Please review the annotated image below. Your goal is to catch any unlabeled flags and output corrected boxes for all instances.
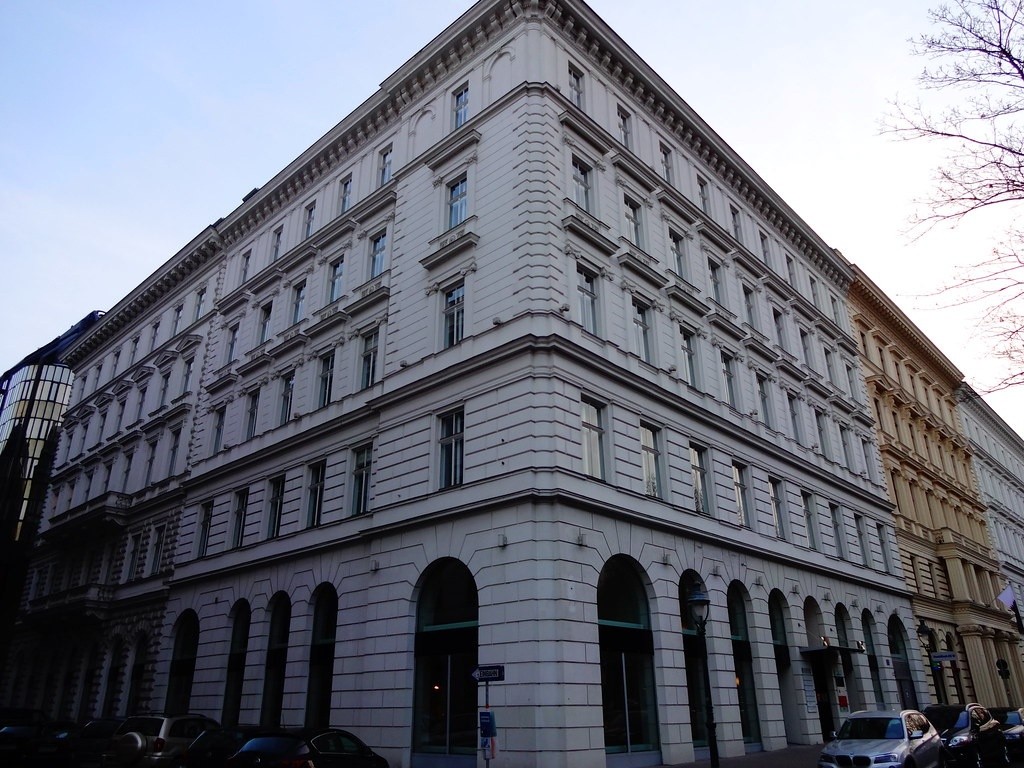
[997,586,1024,638]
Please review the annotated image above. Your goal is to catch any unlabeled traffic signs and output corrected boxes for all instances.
[470,665,506,683]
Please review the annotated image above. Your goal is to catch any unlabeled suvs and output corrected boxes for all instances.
[923,701,1009,768]
[817,708,948,768]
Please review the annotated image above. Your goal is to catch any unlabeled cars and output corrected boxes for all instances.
[988,706,1024,753]
[0,706,391,768]
